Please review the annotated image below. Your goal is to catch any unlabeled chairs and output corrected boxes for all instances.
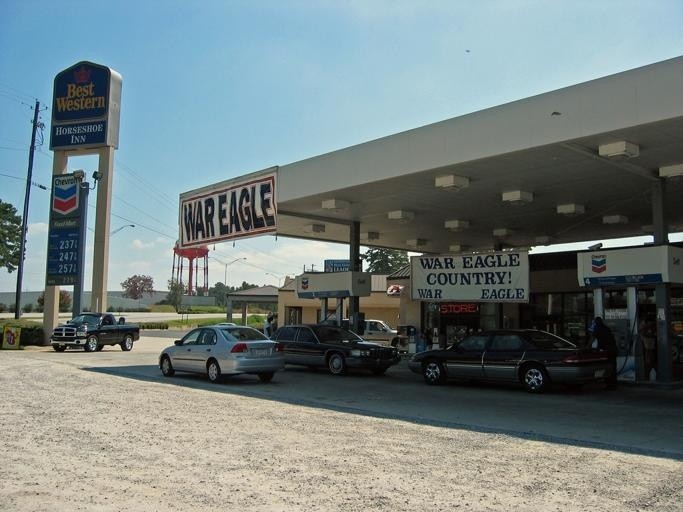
[204,335,214,344]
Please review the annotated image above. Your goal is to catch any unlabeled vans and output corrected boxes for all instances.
[334,319,401,346]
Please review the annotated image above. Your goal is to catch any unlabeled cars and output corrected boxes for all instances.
[270,324,400,375]
[409,328,606,393]
[159,323,284,384]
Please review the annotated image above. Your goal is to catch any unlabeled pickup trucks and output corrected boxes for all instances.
[49,312,139,352]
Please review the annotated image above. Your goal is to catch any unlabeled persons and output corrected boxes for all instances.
[585,317,617,391]
[263,314,273,338]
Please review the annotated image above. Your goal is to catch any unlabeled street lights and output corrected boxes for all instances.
[209,256,247,288]
[86,222,136,239]
[267,271,295,289]
[73,171,103,318]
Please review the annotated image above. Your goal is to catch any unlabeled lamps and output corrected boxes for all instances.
[660,164,682,182]
[491,191,676,246]
[435,174,470,193]
[302,199,472,253]
[598,140,640,162]
